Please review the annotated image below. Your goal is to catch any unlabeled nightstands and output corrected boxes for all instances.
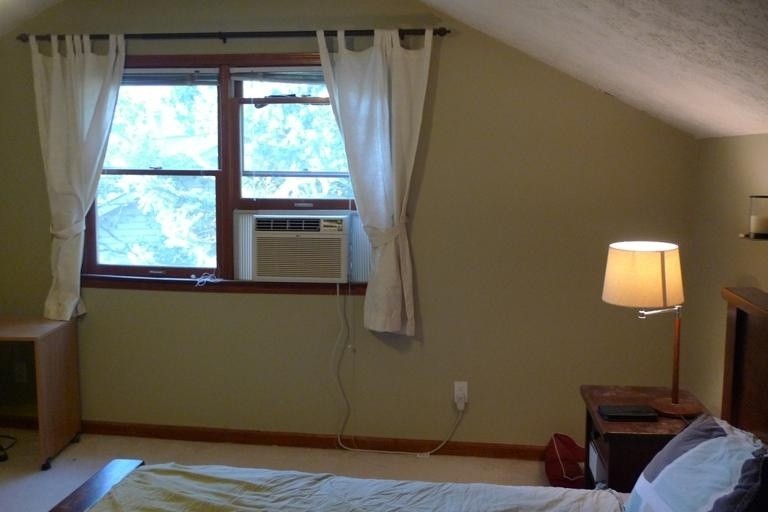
[571,381,714,493]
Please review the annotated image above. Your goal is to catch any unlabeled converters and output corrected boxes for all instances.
[457,391,465,411]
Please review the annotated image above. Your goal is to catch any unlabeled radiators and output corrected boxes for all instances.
[251,213,350,280]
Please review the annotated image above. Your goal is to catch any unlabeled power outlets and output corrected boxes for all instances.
[451,378,468,405]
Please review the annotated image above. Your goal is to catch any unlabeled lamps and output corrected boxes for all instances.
[600,240,707,424]
[745,195,768,239]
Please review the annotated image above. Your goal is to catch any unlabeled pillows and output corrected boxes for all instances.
[618,407,767,512]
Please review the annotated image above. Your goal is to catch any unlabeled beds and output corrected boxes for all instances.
[33,284,767,511]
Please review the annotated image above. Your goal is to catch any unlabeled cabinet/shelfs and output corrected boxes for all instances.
[0,310,84,477]
